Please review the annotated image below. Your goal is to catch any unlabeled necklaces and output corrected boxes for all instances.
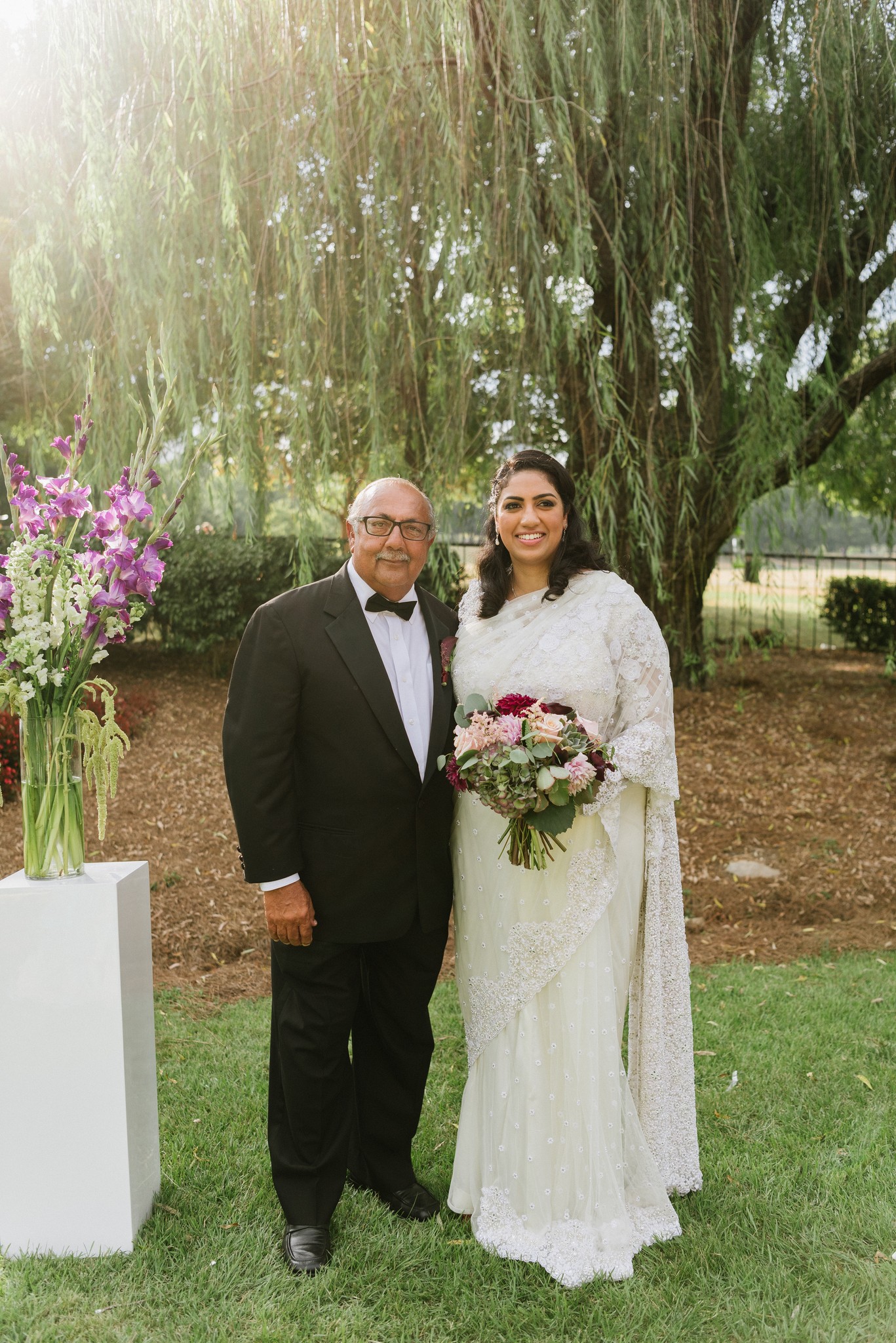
[510,569,544,615]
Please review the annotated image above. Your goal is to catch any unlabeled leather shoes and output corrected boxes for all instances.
[346,1171,441,1222]
[281,1223,331,1277]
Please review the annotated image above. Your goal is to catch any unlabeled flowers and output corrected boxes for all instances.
[1,392,229,880]
[434,692,620,874]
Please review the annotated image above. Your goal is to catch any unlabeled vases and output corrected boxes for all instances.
[17,717,85,880]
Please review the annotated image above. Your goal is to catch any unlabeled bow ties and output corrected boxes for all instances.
[364,592,417,621]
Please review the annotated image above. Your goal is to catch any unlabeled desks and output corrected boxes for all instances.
[0,861,164,1263]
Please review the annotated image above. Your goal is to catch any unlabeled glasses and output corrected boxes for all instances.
[353,515,432,541]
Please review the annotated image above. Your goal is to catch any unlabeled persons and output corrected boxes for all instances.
[202,521,212,534]
[450,448,699,1287]
[744,553,760,583]
[218,476,453,1275]
